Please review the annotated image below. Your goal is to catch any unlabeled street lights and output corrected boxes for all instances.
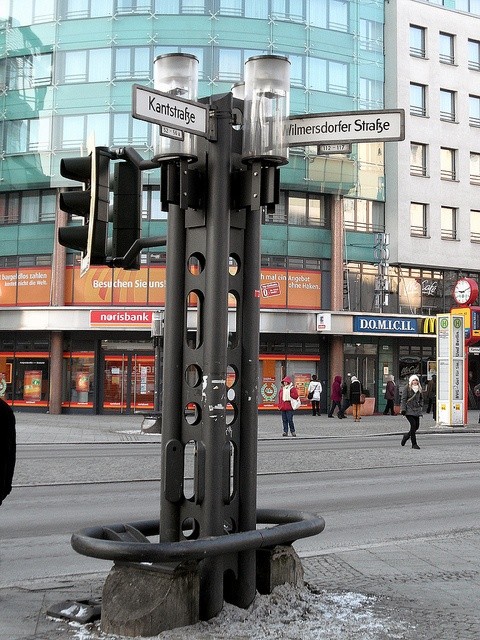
[71,52,326,637]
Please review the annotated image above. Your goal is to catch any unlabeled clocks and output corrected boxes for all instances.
[450,275,477,311]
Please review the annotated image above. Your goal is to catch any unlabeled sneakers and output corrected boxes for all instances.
[282,432,287,435]
[291,431,295,436]
[384,412,390,415]
[392,413,397,416]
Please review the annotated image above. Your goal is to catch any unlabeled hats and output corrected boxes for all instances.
[408,374,422,393]
[431,374,435,376]
[386,374,393,381]
[351,376,357,381]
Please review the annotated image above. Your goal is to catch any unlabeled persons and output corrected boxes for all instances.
[343,372,352,418]
[427,374,438,421]
[307,374,323,413]
[401,375,422,449]
[382,375,399,416]
[277,374,299,436]
[329,374,346,417]
[350,375,366,422]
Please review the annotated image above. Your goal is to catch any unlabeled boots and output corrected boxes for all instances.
[341,412,346,417]
[315,407,320,415]
[400,433,409,446]
[411,436,419,449]
[312,408,315,415]
[327,410,333,417]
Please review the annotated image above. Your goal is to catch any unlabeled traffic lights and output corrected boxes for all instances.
[49,146,112,267]
[107,147,142,269]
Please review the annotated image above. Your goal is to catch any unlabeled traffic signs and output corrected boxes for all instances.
[287,108,405,148]
[131,83,218,141]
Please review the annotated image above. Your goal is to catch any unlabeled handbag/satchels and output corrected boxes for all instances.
[289,385,300,410]
[307,384,319,398]
[359,382,365,403]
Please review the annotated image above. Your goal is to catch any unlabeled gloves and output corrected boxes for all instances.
[402,411,406,416]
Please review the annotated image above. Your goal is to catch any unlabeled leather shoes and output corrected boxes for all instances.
[336,412,341,418]
